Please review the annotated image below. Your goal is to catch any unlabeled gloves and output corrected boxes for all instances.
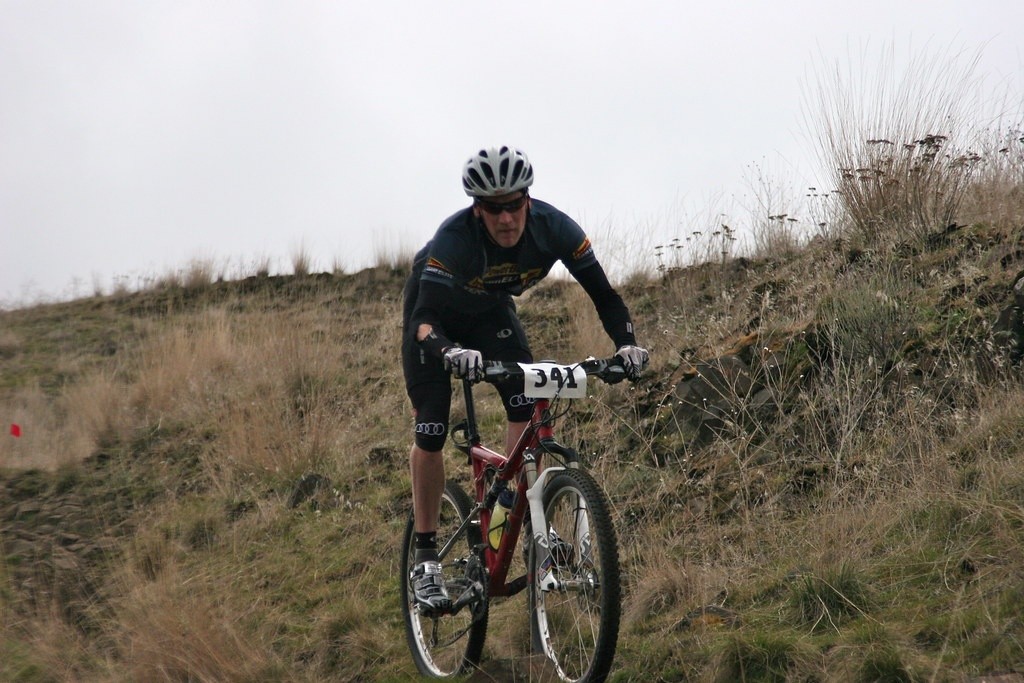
[442,346,485,386]
[613,345,649,384]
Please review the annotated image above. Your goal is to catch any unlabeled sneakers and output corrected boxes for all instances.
[410,560,453,617]
[521,521,575,574]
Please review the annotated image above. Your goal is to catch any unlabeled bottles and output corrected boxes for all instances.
[485,489,515,552]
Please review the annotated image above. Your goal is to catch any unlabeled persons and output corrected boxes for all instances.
[402,146,651,614]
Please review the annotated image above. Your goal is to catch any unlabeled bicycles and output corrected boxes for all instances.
[397,353,630,683]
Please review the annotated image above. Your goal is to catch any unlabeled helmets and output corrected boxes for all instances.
[460,146,533,197]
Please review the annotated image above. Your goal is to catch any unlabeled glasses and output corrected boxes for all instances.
[473,191,529,215]
